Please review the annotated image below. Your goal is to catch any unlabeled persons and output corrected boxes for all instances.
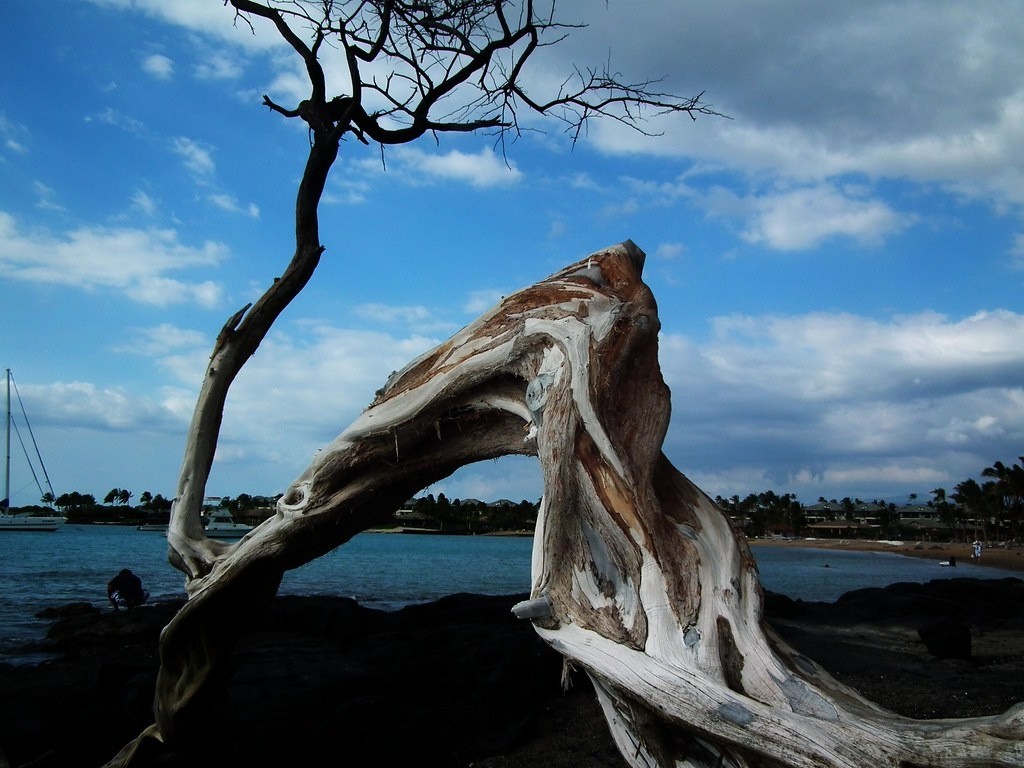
[107,568,151,612]
[949,537,1020,570]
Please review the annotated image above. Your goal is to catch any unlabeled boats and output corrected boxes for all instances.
[201,508,256,539]
[0,508,67,530]
